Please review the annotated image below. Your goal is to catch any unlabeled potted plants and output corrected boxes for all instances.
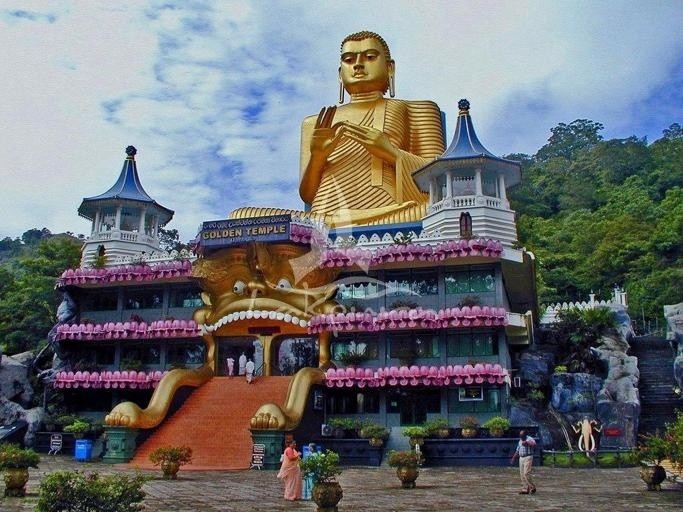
[148,444,192,480]
[326,416,389,447]
[338,350,361,369]
[399,416,450,447]
[62,421,91,439]
[480,415,511,438]
[628,427,678,492]
[297,449,343,512]
[0,441,40,497]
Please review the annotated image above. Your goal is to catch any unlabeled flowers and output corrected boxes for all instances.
[383,449,425,469]
[458,415,479,430]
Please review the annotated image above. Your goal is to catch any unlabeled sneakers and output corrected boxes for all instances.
[519,490,529,494]
[530,486,536,494]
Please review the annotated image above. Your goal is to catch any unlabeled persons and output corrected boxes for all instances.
[227,356,235,379]
[245,358,255,384]
[302,442,320,500]
[239,351,247,376]
[230,29,444,228]
[277,440,303,500]
[510,431,538,494]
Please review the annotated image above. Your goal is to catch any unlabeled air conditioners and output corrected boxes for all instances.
[320,424,332,437]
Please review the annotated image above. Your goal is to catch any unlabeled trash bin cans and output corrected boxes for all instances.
[303,446,323,463]
[76,440,93,461]
